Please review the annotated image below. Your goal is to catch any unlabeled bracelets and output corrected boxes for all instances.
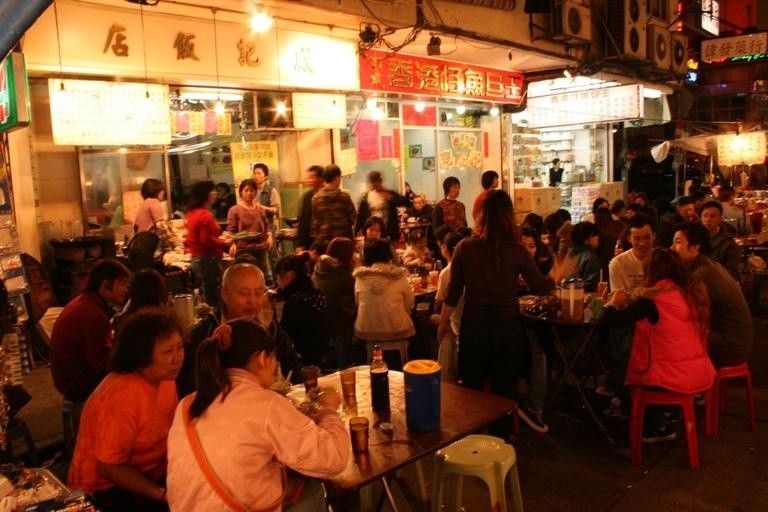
[160,488,166,504]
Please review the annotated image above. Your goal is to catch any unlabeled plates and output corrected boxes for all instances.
[21,466,73,512]
[232,233,262,242]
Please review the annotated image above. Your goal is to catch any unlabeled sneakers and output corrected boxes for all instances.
[642,429,677,443]
[514,402,549,434]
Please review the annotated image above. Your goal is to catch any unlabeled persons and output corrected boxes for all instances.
[660,196,700,231]
[600,247,716,443]
[635,193,649,206]
[403,229,435,275]
[405,183,417,202]
[717,184,744,234]
[624,202,643,220]
[125,232,168,276]
[310,237,356,351]
[432,176,468,235]
[517,227,539,260]
[687,186,711,209]
[436,189,556,443]
[472,171,499,222]
[520,213,553,277]
[549,221,604,293]
[227,180,273,283]
[351,239,417,341]
[434,235,465,385]
[695,200,738,273]
[113,269,174,362]
[548,158,566,188]
[175,263,308,402]
[543,209,572,253]
[266,252,330,366]
[434,225,453,249]
[166,318,350,512]
[186,180,234,315]
[48,259,132,404]
[602,215,657,419]
[211,182,236,218]
[582,198,620,225]
[251,163,281,263]
[669,229,753,405]
[309,170,356,254]
[133,178,167,237]
[612,199,626,217]
[293,165,326,254]
[356,170,411,241]
[411,195,433,220]
[353,215,385,254]
[67,313,185,512]
[593,206,625,241]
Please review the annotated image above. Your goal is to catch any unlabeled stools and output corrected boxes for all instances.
[366,338,408,368]
[435,434,524,511]
[706,363,757,436]
[631,389,699,473]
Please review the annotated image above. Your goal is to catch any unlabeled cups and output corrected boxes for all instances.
[410,277,422,291]
[591,297,604,318]
[271,380,293,395]
[340,369,357,402]
[597,282,608,306]
[301,365,319,393]
[561,277,584,321]
[348,417,370,455]
[403,359,443,434]
[429,271,438,289]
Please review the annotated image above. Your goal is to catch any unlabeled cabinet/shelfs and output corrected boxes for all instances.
[512,128,541,179]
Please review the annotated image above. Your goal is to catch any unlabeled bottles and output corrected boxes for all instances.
[370,344,390,410]
[614,240,623,256]
